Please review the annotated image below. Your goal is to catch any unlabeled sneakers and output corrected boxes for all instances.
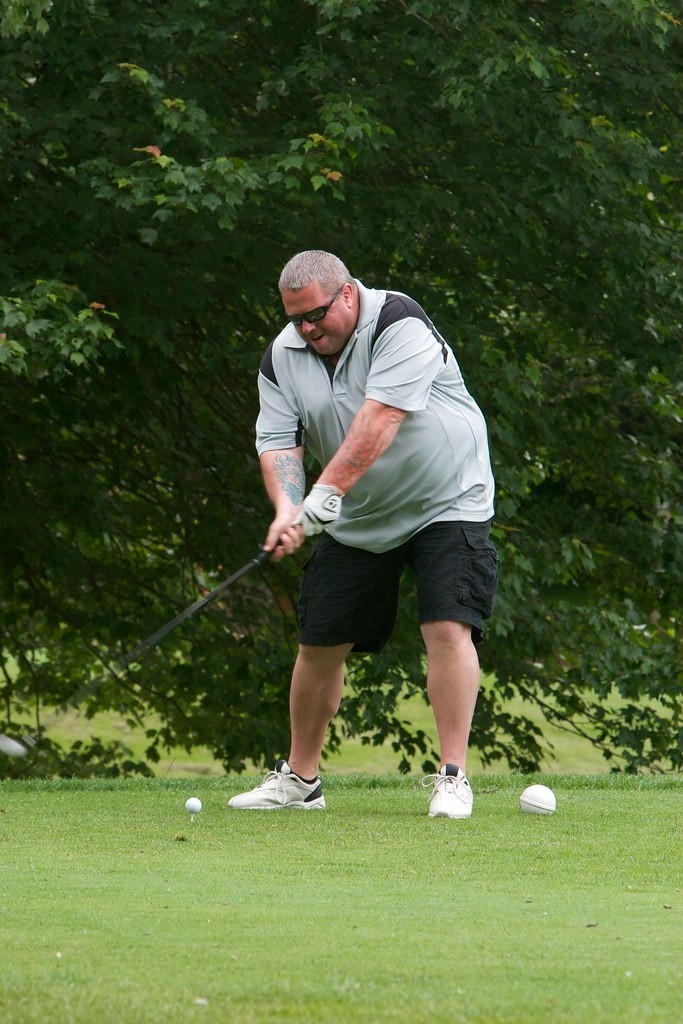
[422,764,474,819]
[227,760,325,811]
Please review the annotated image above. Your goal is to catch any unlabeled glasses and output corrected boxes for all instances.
[285,282,351,326]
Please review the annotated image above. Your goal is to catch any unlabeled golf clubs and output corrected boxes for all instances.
[0,538,283,758]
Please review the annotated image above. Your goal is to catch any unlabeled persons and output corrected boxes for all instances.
[227,250,497,820]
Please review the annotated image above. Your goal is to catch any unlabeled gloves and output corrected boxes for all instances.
[292,483,345,536]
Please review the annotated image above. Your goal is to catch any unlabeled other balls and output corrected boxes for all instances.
[519,784,556,815]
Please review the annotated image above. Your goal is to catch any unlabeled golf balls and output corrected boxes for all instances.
[184,797,202,814]
